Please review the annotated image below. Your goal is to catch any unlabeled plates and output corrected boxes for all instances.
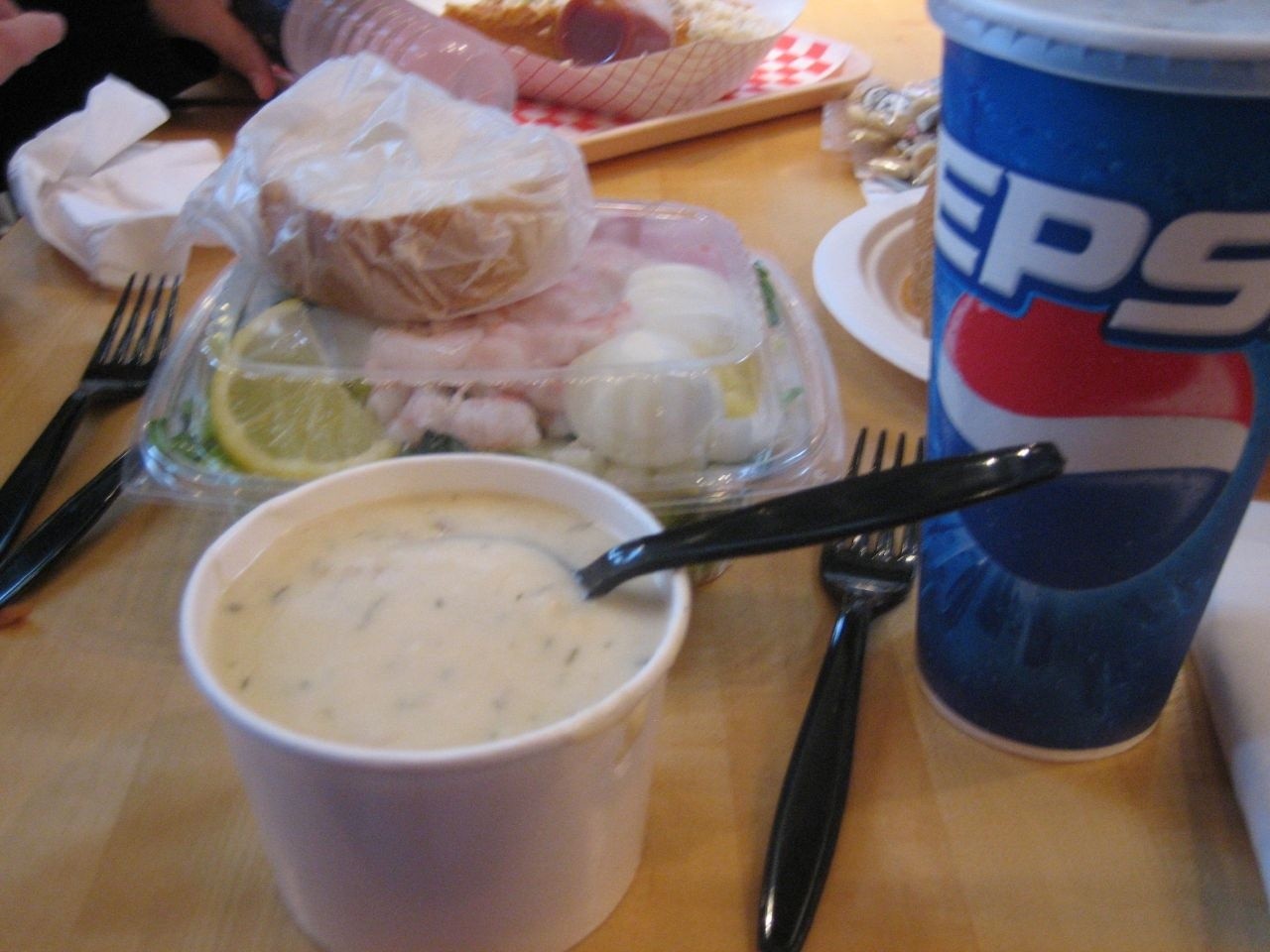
[514,28,875,168]
[811,184,931,384]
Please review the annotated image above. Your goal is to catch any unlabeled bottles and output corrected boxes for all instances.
[226,0,516,115]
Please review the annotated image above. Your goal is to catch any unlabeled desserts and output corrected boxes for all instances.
[439,0,695,70]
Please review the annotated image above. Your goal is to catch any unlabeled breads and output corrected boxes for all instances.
[262,73,585,324]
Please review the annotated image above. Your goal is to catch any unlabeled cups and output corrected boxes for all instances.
[908,0,1270,762]
[177,449,694,952]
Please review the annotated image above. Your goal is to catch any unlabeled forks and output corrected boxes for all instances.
[757,427,926,952]
[0,270,183,559]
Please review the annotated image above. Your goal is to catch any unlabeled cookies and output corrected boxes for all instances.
[282,0,518,114]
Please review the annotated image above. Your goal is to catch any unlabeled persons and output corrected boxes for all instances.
[0,0,300,197]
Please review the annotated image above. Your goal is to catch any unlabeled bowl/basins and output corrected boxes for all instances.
[120,197,848,586]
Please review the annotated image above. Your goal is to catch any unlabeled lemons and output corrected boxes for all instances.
[208,298,396,478]
